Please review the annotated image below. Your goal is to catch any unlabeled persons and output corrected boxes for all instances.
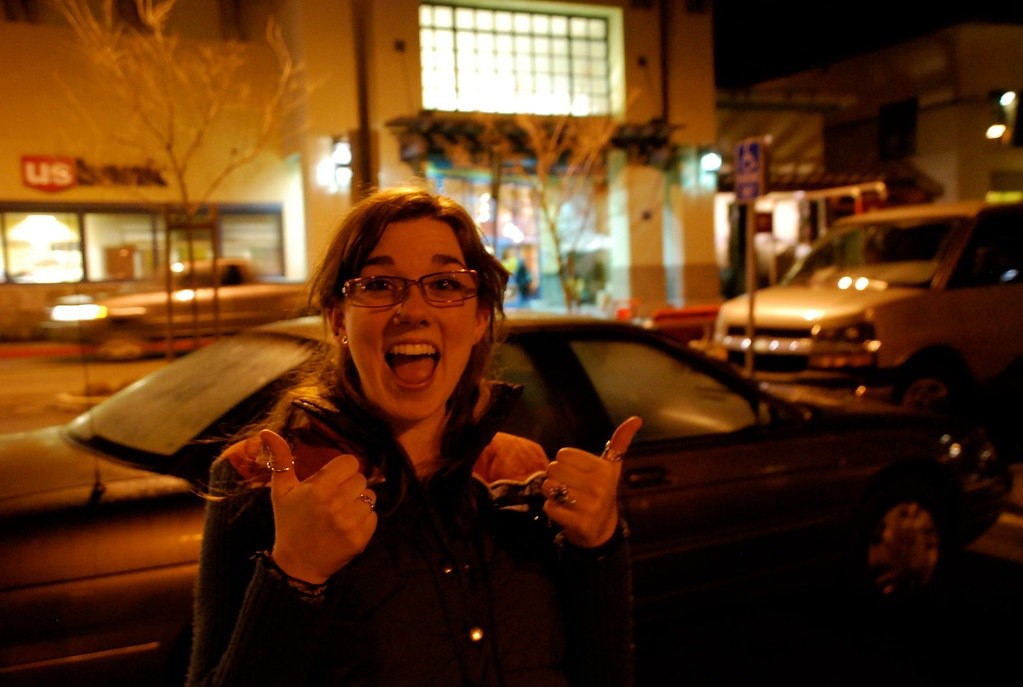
[183,186,642,687]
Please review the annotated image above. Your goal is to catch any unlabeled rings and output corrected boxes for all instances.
[605,440,624,463]
[548,484,576,506]
[359,494,374,512]
[266,459,294,472]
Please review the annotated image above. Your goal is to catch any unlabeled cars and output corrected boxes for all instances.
[1,314,1013,687]
[45,261,323,364]
[711,200,1023,414]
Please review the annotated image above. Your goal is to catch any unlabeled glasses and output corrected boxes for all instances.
[341,268,480,309]
